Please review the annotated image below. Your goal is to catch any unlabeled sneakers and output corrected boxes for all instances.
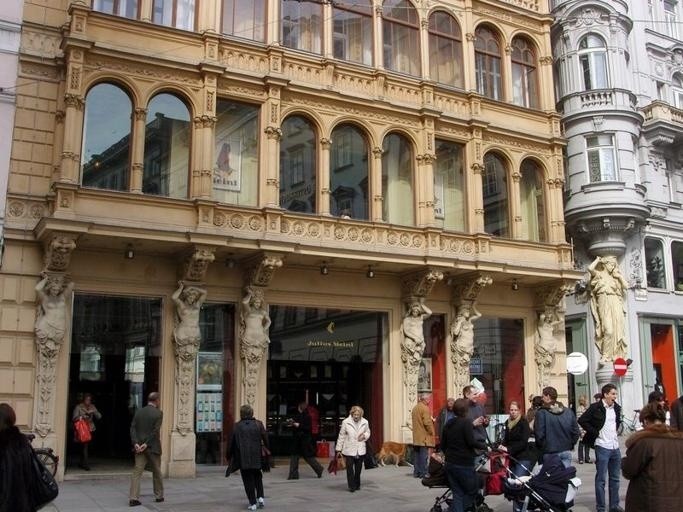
[598,505,625,512]
[579,460,592,464]
[247,497,265,510]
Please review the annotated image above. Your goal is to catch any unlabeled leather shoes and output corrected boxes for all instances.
[130,498,164,506]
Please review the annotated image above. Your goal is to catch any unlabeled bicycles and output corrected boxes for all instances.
[23,433,59,478]
[616,409,644,436]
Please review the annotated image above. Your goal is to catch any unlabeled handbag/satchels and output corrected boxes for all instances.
[328,451,346,475]
[262,444,274,472]
[72,416,92,443]
[486,453,509,494]
[30,446,58,511]
[315,438,335,458]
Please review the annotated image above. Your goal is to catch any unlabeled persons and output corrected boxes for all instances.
[412,384,683,512]
[0,402,37,511]
[237,285,271,362]
[398,298,433,361]
[130,392,164,506]
[287,402,323,480]
[35,270,75,357]
[72,393,102,470]
[587,254,629,362]
[417,361,431,389]
[450,299,482,366]
[225,404,270,512]
[216,143,233,175]
[335,399,371,492]
[171,280,207,346]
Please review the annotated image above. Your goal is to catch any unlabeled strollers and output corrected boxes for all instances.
[422,448,509,511]
[484,448,582,512]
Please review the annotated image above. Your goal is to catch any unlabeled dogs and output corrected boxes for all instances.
[376,441,406,468]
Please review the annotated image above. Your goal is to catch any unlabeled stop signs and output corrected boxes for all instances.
[614,358,627,376]
